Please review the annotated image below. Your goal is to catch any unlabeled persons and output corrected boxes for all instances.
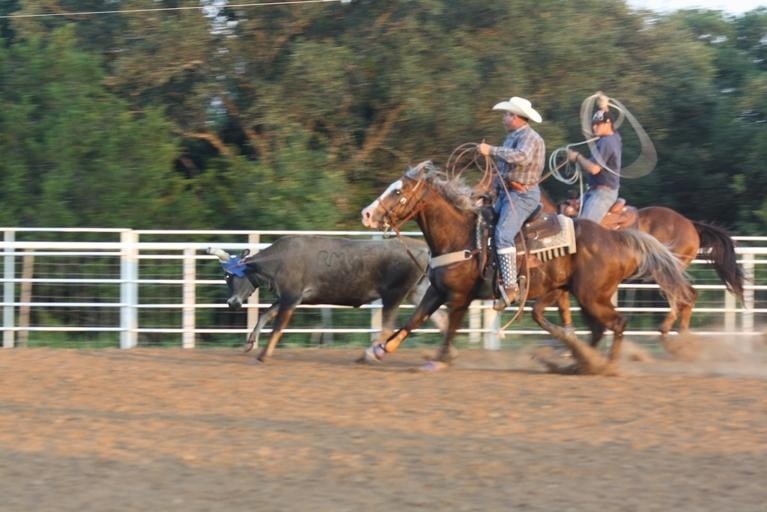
[476,96,546,311]
[574,90,623,226]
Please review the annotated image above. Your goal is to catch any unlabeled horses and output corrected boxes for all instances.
[359,159,699,377]
[468,168,760,364]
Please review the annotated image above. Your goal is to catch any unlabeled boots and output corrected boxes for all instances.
[492,246,521,312]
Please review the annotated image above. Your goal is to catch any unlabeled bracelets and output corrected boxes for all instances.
[573,153,579,160]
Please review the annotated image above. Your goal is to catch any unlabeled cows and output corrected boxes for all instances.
[204,234,460,364]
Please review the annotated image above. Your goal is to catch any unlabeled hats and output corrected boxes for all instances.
[492,94,544,125]
[590,110,614,124]
